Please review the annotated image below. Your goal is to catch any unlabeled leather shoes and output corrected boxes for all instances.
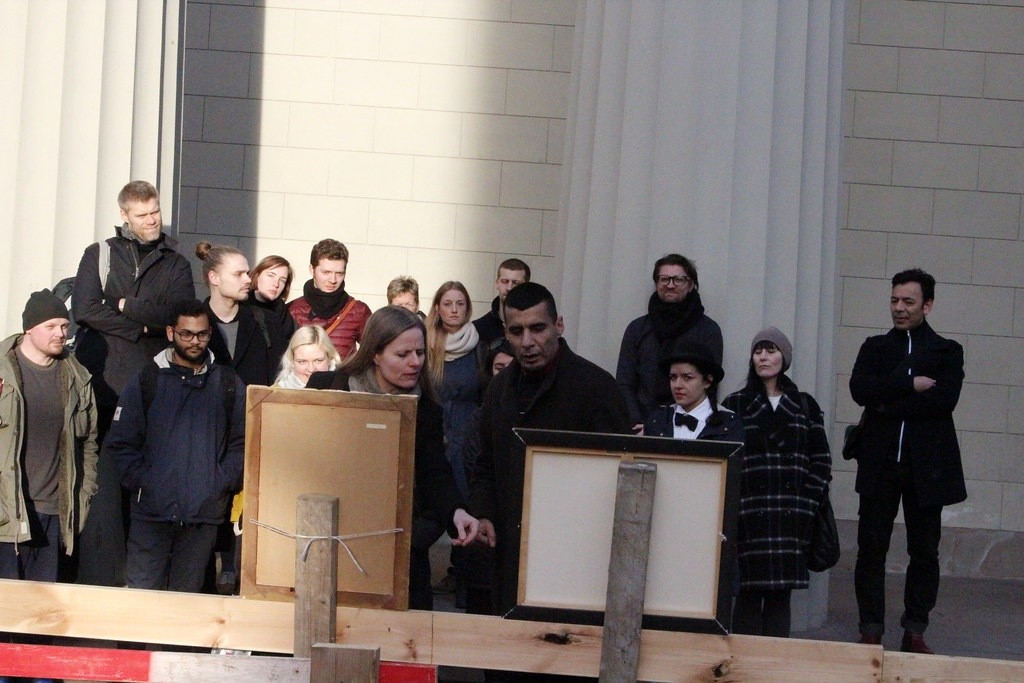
[859,631,882,648]
[901,628,934,656]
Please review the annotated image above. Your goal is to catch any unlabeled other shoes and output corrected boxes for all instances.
[430,570,456,594]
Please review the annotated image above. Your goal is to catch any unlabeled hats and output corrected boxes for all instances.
[22,289,71,331]
[664,342,724,385]
[751,326,792,372]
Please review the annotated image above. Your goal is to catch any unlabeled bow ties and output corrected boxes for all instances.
[675,412,700,432]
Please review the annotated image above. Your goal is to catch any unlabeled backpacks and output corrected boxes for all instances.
[53,239,113,348]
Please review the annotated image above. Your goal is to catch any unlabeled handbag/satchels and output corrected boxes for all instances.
[842,424,863,461]
[803,496,840,573]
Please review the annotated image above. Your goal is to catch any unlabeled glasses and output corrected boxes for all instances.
[656,274,692,286]
[173,329,211,343]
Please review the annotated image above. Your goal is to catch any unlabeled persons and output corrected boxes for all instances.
[849,269,969,654]
[384,258,530,606]
[616,252,745,633]
[306,304,481,610]
[195,238,372,595]
[71,180,246,652]
[463,282,632,621]
[0,288,99,683]
[719,325,832,637]
